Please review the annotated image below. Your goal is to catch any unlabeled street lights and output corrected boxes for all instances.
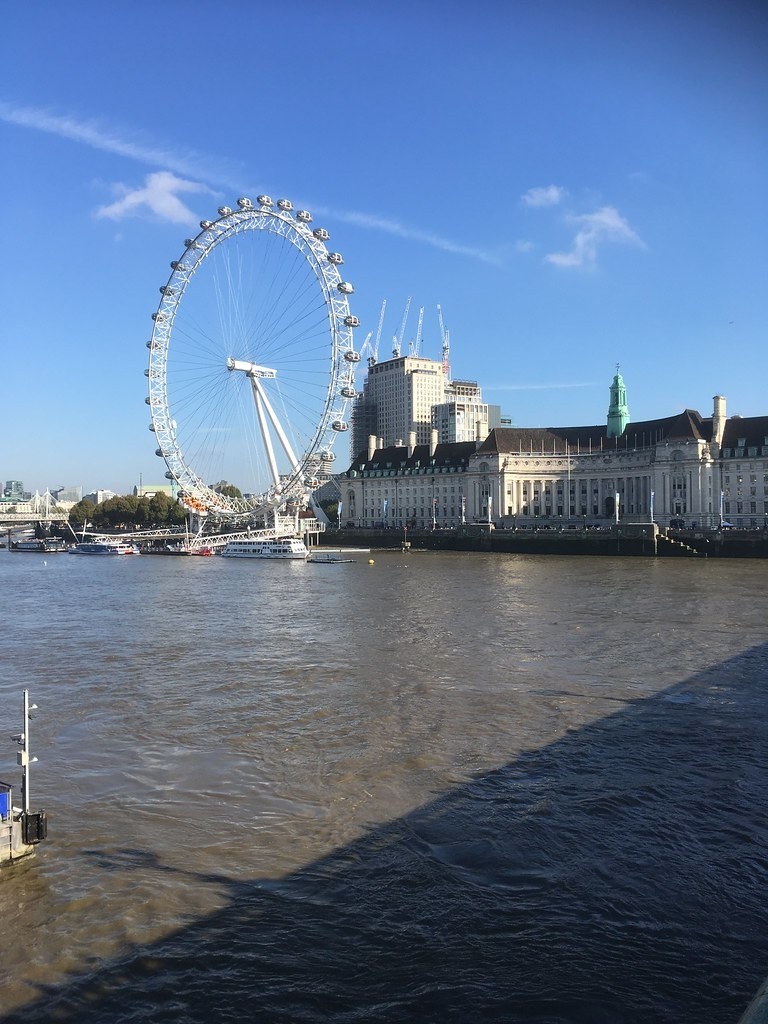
[395,479,399,529]
[719,490,724,526]
[431,476,436,531]
[649,487,654,523]
[362,481,365,528]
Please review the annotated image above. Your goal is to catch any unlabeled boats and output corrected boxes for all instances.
[68,535,142,557]
[220,539,313,559]
[199,546,212,556]
